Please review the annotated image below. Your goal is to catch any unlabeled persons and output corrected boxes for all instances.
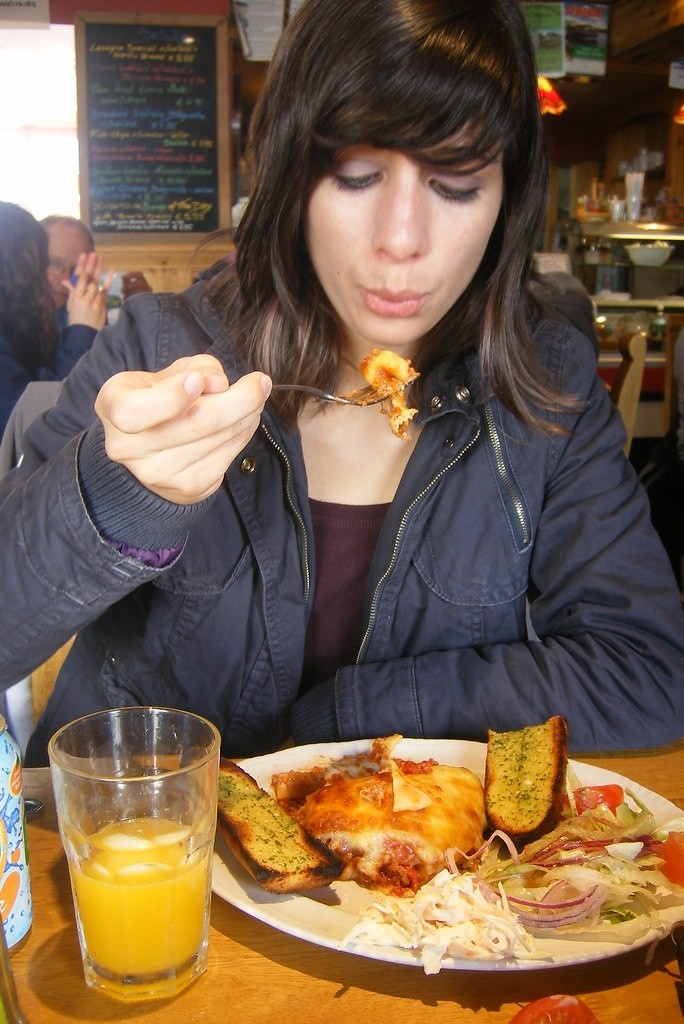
[0,201,116,445]
[39,215,95,309]
[0,0,684,769]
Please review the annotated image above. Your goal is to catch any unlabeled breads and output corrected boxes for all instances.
[484,714,568,844]
[179,746,346,894]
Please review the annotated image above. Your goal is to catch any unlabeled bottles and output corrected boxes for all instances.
[1,716,31,958]
[588,178,604,212]
[578,198,584,217]
[596,312,667,351]
[577,239,612,263]
[646,192,657,219]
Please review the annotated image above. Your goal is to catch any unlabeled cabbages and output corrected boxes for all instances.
[478,771,684,937]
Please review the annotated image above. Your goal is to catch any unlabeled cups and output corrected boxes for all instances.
[607,198,625,223]
[625,168,644,221]
[49,707,220,1000]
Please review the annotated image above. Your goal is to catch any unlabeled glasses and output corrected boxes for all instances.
[46,260,74,277]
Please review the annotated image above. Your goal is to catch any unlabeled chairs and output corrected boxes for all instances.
[661,318,684,436]
[610,332,647,459]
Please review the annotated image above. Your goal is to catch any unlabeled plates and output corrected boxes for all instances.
[208,738,657,970]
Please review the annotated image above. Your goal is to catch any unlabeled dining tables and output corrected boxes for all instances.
[0,737,684,1024]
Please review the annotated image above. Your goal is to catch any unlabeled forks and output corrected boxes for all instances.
[271,371,421,406]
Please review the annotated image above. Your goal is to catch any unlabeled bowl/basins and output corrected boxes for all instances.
[625,244,674,266]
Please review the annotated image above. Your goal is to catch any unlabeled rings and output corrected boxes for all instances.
[98,286,105,293]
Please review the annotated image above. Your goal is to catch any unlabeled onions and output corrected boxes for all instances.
[445,831,667,928]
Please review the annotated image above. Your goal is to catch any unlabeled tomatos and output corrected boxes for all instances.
[661,830,684,887]
[560,784,624,816]
